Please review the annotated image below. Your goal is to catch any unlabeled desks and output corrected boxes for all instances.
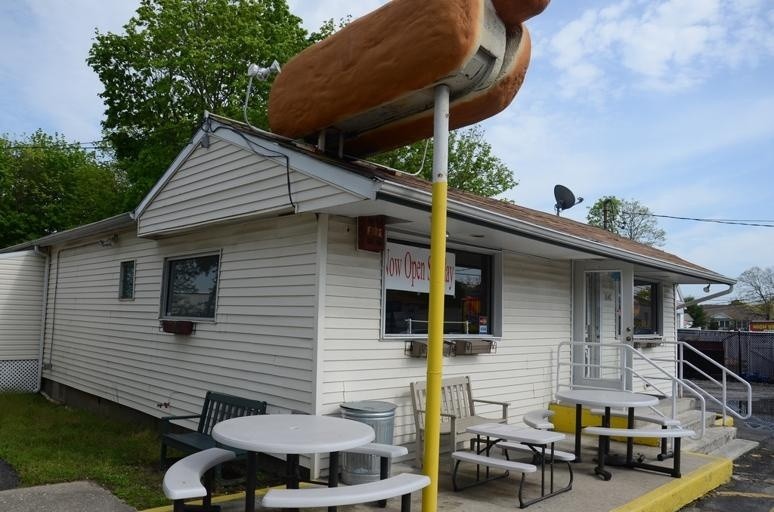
[212,414,376,489]
[467,421,566,445]
[553,388,662,450]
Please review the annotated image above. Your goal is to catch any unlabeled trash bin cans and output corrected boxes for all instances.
[339,400,398,486]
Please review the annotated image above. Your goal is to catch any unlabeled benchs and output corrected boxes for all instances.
[261,473,431,511]
[451,448,537,494]
[478,436,577,475]
[583,424,696,450]
[294,442,409,507]
[159,448,236,510]
[589,409,682,429]
[525,409,556,430]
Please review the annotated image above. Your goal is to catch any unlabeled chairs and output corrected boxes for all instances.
[158,390,267,494]
[408,375,511,477]
